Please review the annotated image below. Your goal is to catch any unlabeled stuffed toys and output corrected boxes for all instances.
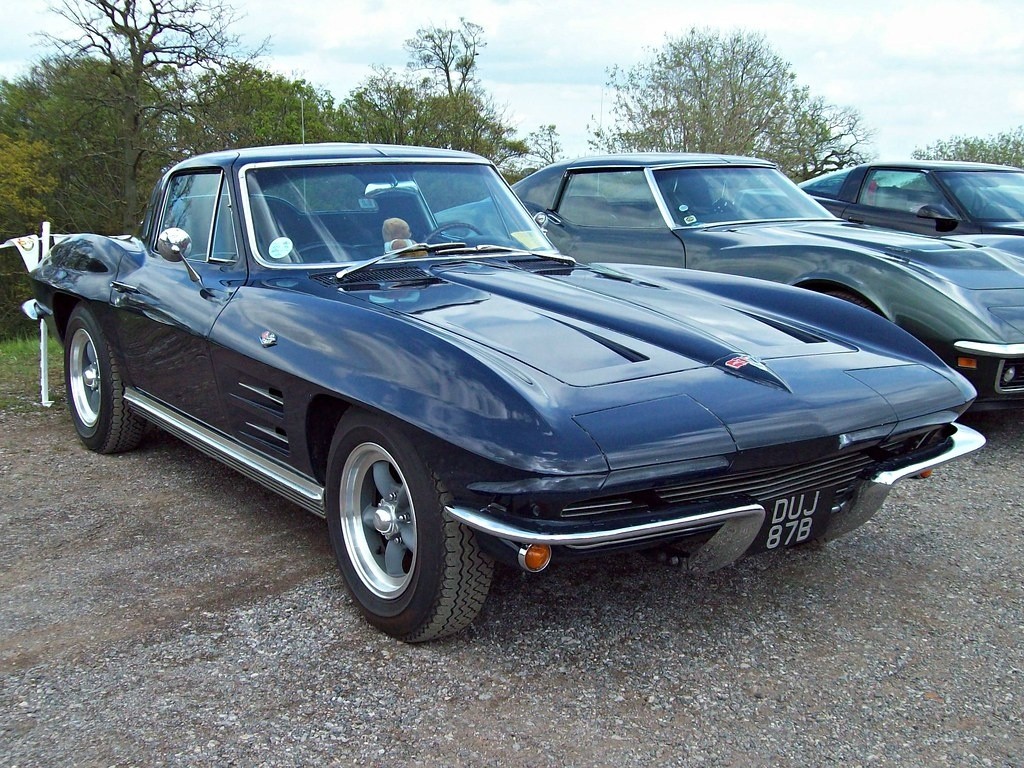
[382,218,417,254]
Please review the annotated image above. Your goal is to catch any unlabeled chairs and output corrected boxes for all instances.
[652,174,715,230]
[567,195,616,229]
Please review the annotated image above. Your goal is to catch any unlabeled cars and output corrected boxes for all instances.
[25,142,987,646]
[730,157,1024,265]
[423,151,1024,423]
